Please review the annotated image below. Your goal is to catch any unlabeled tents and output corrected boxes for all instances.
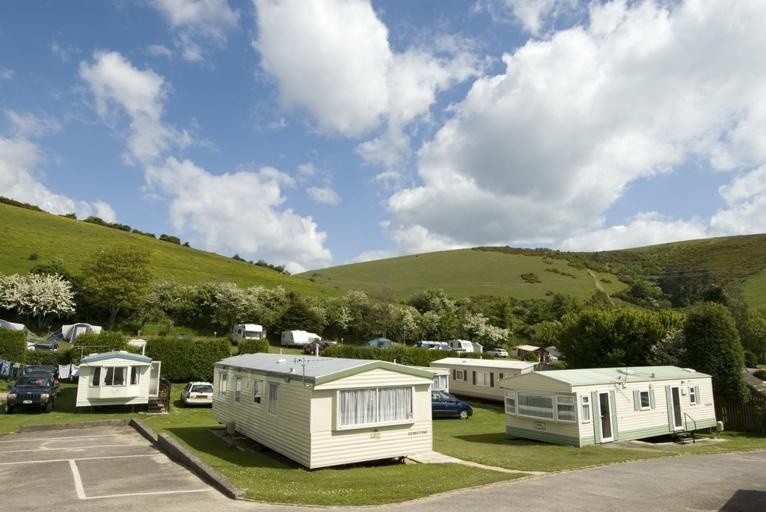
[363,336,391,346]
[47,322,104,342]
[415,340,449,350]
[0,319,36,351]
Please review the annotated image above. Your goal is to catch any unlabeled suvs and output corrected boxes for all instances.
[6,371,54,415]
[486,349,509,358]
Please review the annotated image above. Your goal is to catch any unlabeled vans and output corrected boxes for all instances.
[281,330,320,349]
[231,323,263,347]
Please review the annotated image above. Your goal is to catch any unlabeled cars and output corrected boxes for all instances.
[305,342,330,355]
[181,382,213,406]
[432,390,473,419]
